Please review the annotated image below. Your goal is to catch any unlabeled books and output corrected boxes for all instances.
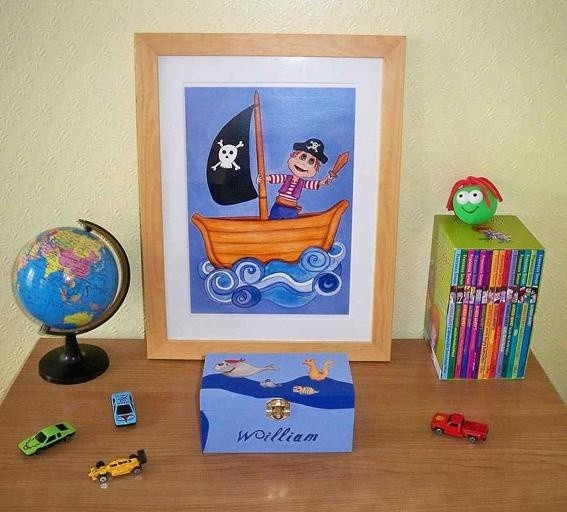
[424,213,546,382]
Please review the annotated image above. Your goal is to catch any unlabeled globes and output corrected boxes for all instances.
[11,219,130,385]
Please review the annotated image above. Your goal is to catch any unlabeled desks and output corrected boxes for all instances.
[2,338,567,511]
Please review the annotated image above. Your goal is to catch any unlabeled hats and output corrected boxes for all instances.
[293,139,327,164]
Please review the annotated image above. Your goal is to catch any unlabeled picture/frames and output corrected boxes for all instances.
[133,32,407,364]
